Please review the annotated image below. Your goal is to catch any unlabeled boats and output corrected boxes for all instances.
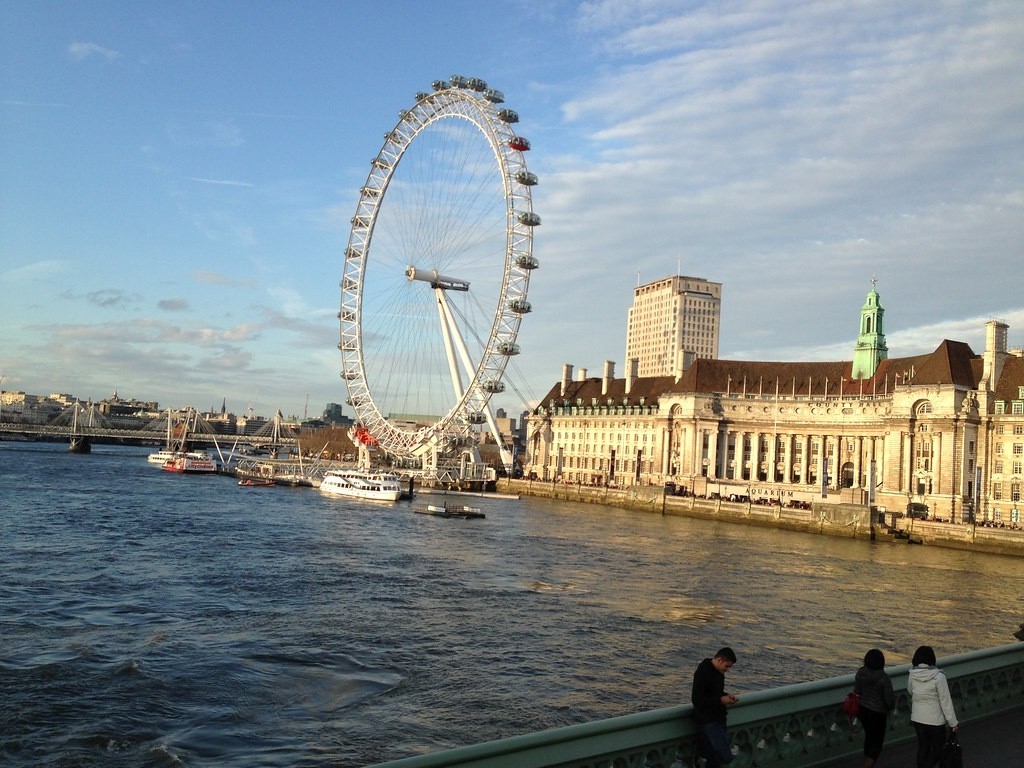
[237,481,277,486]
[147,448,176,468]
[69,436,91,455]
[319,470,402,501]
[162,453,224,475]
[408,501,485,518]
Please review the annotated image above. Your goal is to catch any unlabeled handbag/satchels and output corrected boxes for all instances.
[938,743,963,768]
[843,691,860,716]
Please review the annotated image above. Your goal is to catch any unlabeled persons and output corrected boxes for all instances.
[679,491,812,511]
[521,475,619,489]
[921,515,1022,531]
[692,647,739,768]
[854,649,895,768]
[906,645,959,768]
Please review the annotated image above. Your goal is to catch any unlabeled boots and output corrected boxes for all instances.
[863,756,878,768]
